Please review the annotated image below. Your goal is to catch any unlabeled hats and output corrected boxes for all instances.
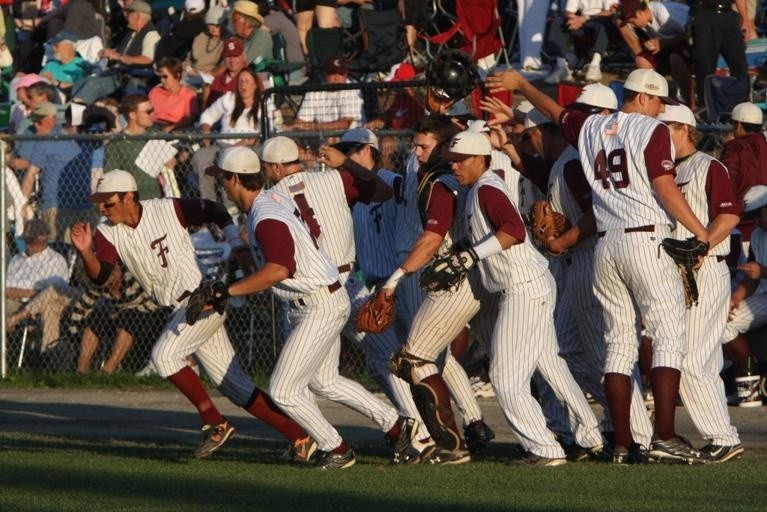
[739,185,766,220]
[18,219,51,243]
[205,145,262,176]
[257,136,300,164]
[89,168,138,203]
[435,129,493,162]
[328,126,379,153]
[514,69,764,140]
[123,0,264,58]
[325,56,348,76]
[13,30,77,121]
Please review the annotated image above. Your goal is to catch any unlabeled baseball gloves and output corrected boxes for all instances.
[418,251,469,292]
[533,201,569,244]
[185,280,228,325]
[661,237,708,266]
[356,289,393,334]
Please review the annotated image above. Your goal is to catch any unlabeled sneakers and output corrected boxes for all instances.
[469,376,495,398]
[545,62,573,84]
[585,62,602,81]
[320,446,357,471]
[286,435,317,463]
[193,416,237,461]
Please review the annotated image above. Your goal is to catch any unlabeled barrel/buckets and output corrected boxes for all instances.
[735,376,762,408]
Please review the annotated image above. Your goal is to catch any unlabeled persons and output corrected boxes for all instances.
[70,68,745,468]
[1,1,766,404]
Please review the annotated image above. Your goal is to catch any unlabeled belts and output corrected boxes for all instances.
[717,256,724,262]
[168,291,191,312]
[598,225,654,237]
[290,281,342,308]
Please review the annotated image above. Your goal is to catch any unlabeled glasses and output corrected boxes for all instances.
[138,108,155,115]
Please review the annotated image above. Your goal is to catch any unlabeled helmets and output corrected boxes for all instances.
[424,47,480,115]
